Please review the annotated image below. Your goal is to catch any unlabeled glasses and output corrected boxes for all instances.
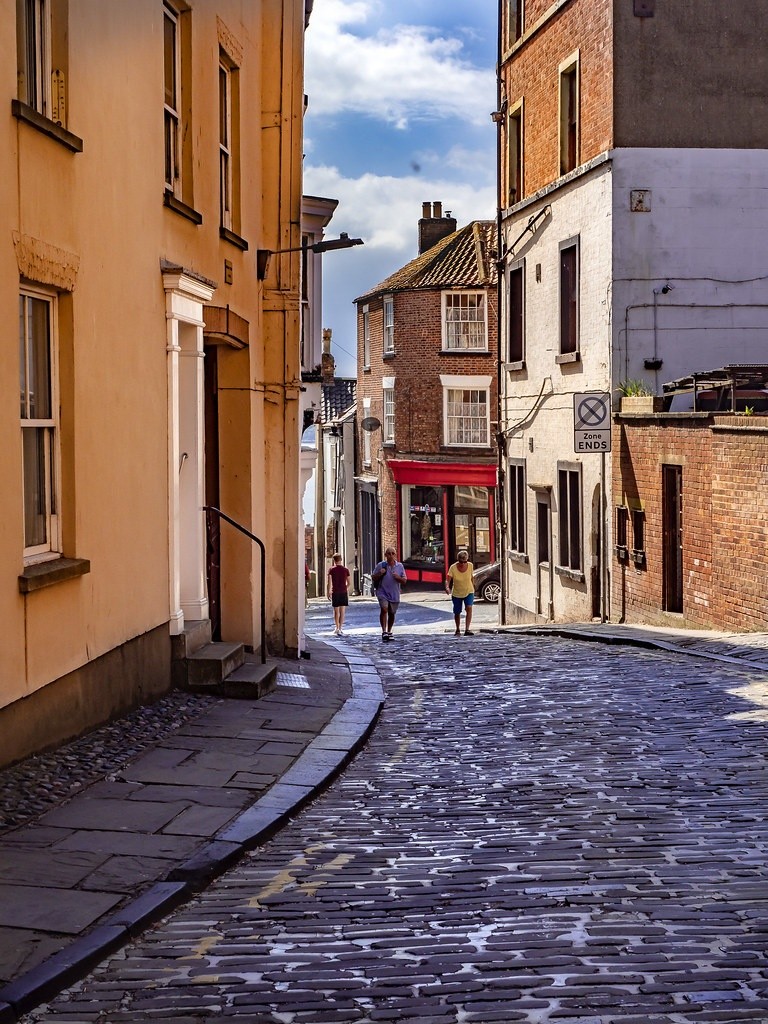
[459,560,467,563]
[388,552,395,555]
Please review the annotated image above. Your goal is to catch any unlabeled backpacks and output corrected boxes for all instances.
[371,561,387,596]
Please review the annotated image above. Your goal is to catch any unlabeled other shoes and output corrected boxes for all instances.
[464,630,474,636]
[454,631,461,636]
[382,633,389,641]
[389,633,394,640]
[334,630,341,634]
[340,629,343,634]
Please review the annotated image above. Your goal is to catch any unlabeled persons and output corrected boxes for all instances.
[445,550,475,636]
[305,561,311,609]
[326,553,351,635]
[371,546,407,641]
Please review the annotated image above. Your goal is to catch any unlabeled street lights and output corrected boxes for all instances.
[328,420,360,597]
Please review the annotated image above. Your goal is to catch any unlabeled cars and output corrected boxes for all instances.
[472,559,501,603]
